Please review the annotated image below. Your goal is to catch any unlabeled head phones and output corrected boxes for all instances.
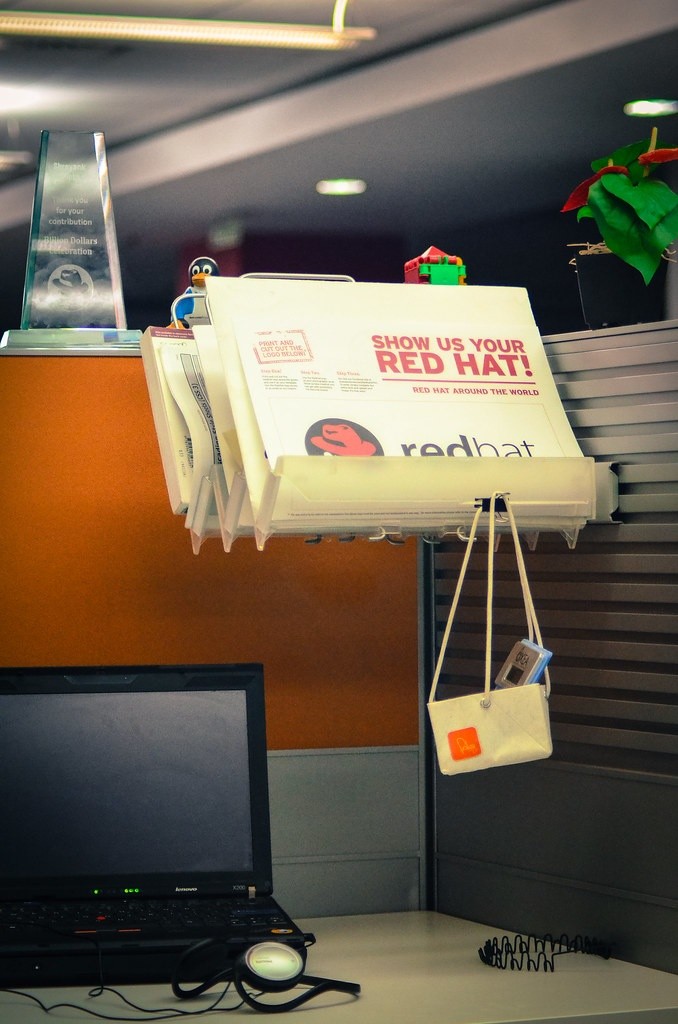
[171,929,361,1015]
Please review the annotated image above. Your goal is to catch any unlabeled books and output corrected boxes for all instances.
[137,321,198,515]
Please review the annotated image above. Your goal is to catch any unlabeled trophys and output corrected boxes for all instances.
[0,128,145,349]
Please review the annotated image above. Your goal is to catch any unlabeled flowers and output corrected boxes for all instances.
[560,126,678,288]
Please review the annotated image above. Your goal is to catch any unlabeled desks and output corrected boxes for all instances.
[0,910,678,1024]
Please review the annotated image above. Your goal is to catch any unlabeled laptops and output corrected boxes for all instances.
[0,663,306,990]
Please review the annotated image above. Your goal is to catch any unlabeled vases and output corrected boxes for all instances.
[574,241,665,329]
